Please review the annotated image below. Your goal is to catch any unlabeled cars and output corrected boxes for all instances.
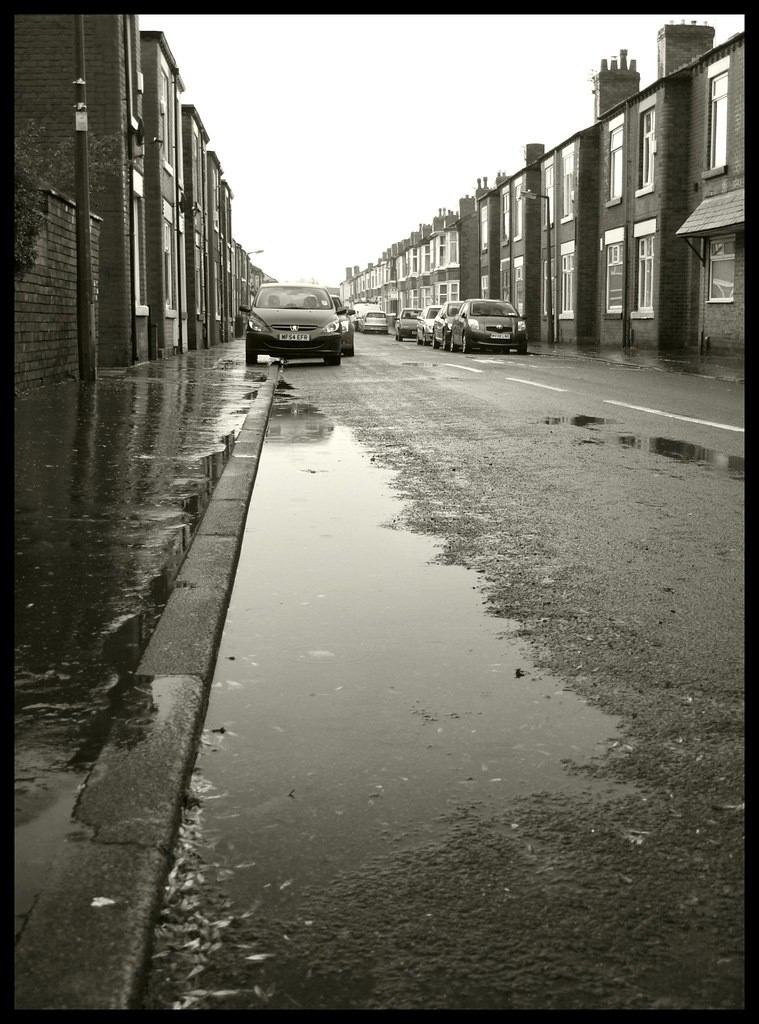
[238,285,357,366]
[363,299,530,353]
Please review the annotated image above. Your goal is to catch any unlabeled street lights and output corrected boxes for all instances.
[245,249,264,312]
[520,190,554,345]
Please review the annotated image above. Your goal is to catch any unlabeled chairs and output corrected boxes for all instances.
[405,313,411,319]
[268,295,279,308]
[304,296,317,308]
[429,311,438,318]
[450,308,458,316]
